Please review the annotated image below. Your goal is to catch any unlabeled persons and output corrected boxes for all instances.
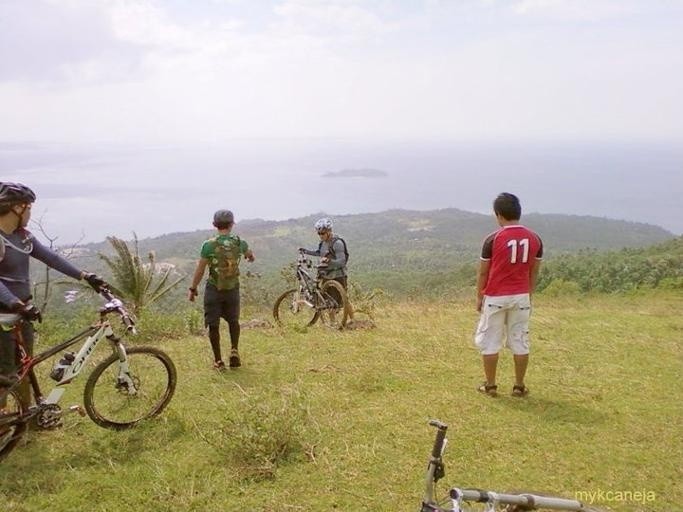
[187,209,255,369]
[298,217,355,325]
[473,192,543,397]
[0,182,114,447]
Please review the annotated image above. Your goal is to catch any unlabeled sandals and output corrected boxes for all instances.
[511,382,529,398]
[476,382,498,397]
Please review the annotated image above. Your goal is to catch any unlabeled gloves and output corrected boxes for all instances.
[83,271,105,294]
[19,302,43,324]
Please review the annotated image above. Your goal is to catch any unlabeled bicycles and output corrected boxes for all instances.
[0,282,179,464]
[418,418,585,512]
[273,248,350,330]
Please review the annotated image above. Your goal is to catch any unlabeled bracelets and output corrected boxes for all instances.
[189,288,196,292]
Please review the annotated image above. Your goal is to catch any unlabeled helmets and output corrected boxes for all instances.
[314,218,332,232]
[0,181,36,212]
[213,209,233,226]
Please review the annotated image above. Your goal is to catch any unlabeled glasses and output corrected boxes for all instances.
[318,231,326,235]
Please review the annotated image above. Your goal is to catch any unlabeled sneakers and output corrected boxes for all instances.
[213,360,225,371]
[229,347,241,368]
